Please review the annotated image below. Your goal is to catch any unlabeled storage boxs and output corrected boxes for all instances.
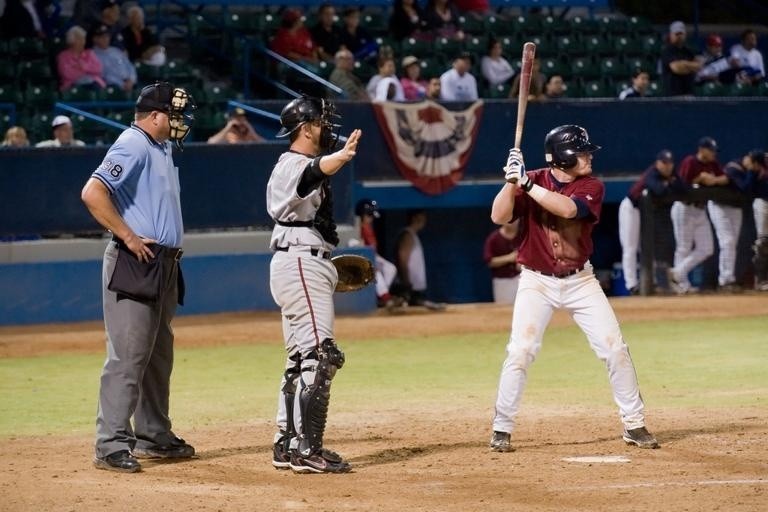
[612,263,639,296]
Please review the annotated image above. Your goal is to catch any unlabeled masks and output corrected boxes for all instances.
[151,52,167,66]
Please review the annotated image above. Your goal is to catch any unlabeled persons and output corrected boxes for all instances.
[82,82,196,475]
[619,136,768,298]
[485,223,520,303]
[490,123,660,453]
[264,93,363,475]
[355,197,426,310]
[1,0,768,143]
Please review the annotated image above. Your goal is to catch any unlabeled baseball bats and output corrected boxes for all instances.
[514,43,536,150]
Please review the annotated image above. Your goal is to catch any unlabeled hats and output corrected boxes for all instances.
[653,136,767,166]
[402,49,472,65]
[51,114,72,126]
[225,108,247,120]
[671,22,683,35]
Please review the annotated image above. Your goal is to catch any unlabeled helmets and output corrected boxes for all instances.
[358,197,380,216]
[276,96,341,151]
[545,123,600,170]
[139,82,196,153]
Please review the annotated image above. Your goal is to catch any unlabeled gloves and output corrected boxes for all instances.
[504,148,533,190]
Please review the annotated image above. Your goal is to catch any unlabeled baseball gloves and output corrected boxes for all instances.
[331,253,376,293]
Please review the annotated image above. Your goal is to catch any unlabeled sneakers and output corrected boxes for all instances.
[272,442,351,474]
[626,267,767,294]
[622,426,659,449]
[133,440,195,460]
[94,452,142,473]
[490,429,511,452]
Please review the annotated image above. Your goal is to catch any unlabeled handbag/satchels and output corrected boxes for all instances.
[107,242,160,300]
